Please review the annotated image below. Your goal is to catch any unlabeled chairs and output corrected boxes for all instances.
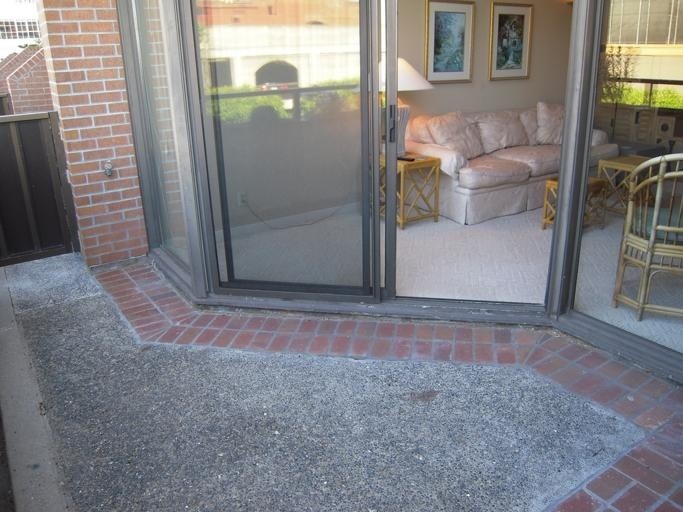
[611,153,683,321]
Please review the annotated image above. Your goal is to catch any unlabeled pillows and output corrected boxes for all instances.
[414,101,565,159]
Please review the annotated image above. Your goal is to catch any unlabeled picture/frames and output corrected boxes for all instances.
[424,0,476,84]
[488,0,534,80]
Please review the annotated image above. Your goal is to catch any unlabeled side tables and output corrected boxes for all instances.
[370,152,441,229]
[616,142,666,188]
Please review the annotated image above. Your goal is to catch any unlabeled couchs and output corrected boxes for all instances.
[404,110,620,226]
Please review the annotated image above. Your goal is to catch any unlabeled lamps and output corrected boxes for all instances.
[353,55,436,156]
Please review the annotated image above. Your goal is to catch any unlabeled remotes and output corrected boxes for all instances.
[397,157,415,161]
